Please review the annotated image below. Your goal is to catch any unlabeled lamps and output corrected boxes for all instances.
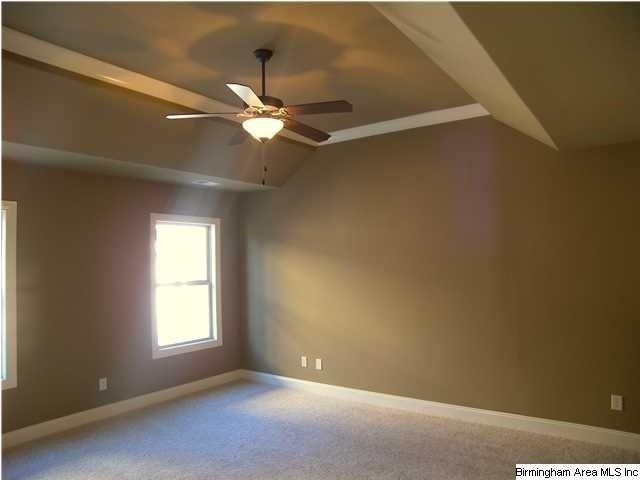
[242,116,284,187]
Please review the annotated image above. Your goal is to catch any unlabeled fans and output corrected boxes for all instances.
[165,50,352,143]
